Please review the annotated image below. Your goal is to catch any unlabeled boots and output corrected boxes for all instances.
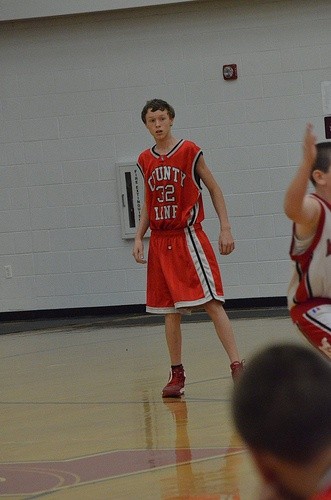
[162,364,186,397]
[230,360,244,388]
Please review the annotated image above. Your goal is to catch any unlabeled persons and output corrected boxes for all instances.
[132,99,245,396]
[285,124,330,361]
[234,344,331,500]
